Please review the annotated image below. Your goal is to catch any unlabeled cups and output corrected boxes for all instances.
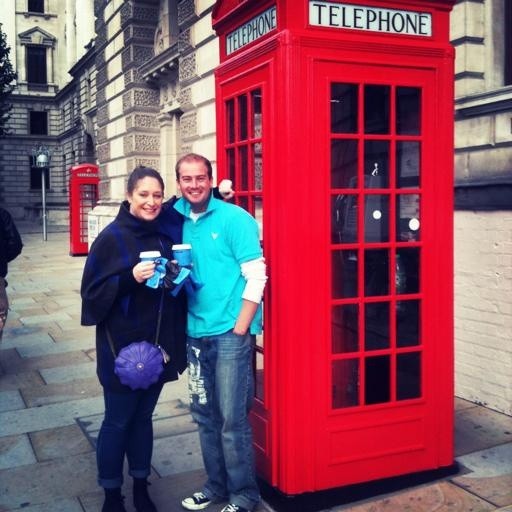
[139,251,163,285]
[171,243,192,281]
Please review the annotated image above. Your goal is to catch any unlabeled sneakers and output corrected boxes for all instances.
[181,483,212,510]
[101,481,125,511]
[133,481,157,511]
[220,502,250,512]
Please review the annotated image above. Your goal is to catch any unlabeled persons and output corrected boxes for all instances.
[0,201,24,348]
[77,165,235,510]
[170,151,268,511]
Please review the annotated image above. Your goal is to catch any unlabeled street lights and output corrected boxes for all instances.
[35,143,51,242]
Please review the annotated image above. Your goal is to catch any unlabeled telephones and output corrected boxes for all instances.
[334,194,359,271]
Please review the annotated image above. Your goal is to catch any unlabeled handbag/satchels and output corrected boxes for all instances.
[113,341,170,392]
